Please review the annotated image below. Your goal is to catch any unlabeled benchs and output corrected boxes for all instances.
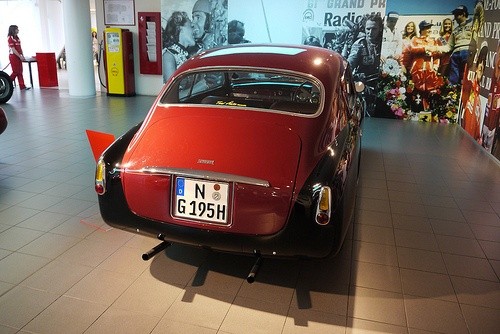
[200,94,321,114]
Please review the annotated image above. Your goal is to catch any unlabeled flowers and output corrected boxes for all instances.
[379,68,459,125]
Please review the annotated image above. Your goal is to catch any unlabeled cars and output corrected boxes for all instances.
[94,44,364,284]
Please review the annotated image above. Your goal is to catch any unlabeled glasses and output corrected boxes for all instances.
[479,57,487,65]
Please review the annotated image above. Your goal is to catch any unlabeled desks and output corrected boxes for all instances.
[14,58,37,89]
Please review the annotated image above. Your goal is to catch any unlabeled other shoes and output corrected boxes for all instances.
[22,87,31,90]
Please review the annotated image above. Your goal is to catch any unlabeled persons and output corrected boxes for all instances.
[7,25,30,90]
[91,28,98,61]
[304,4,500,155]
[162,0,254,88]
[57,48,66,69]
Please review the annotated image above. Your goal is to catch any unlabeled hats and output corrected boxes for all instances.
[478,41,489,56]
[387,12,401,19]
[452,5,468,14]
[419,20,435,27]
[192,0,215,14]
